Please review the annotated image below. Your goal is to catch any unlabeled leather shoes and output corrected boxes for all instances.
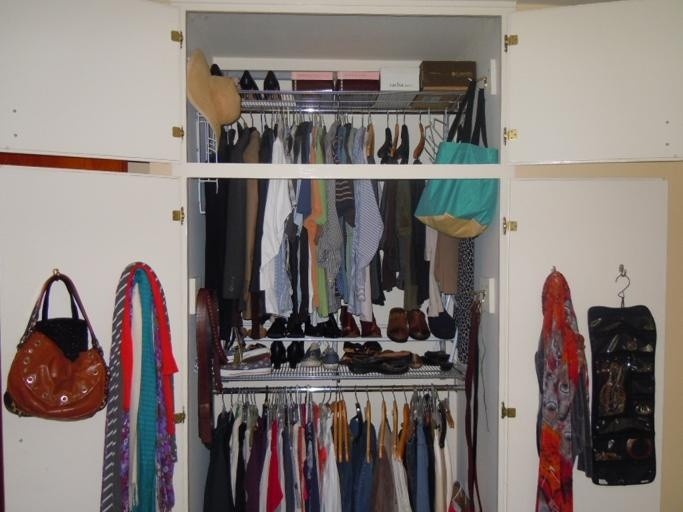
[220,306,455,374]
[242,70,283,100]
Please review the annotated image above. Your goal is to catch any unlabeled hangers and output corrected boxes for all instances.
[220,380,455,463]
[227,90,468,160]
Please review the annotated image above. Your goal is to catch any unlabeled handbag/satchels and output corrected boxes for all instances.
[4,273,110,421]
[414,81,498,238]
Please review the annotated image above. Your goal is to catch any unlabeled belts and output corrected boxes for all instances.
[196,287,228,448]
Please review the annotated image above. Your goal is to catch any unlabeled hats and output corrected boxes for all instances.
[186,47,240,147]
